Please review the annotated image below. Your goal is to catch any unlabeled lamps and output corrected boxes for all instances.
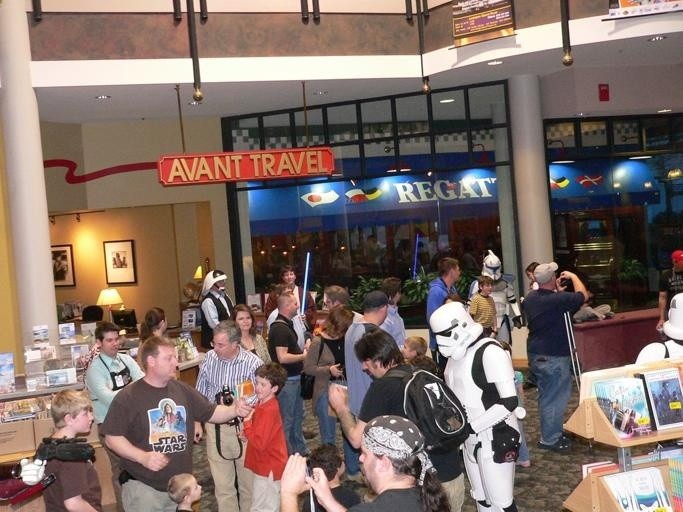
[97,287,123,323]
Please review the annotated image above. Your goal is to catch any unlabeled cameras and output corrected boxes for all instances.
[560,278,574,292]
[305,457,315,484]
[215,384,234,405]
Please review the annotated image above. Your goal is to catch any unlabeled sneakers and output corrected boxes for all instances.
[346,471,365,484]
[562,429,574,440]
[537,435,572,450]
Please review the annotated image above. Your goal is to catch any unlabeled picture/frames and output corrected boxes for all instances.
[102,239,136,284]
[51,244,75,287]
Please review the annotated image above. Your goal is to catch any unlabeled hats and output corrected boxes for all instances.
[533,262,557,284]
[671,250,683,265]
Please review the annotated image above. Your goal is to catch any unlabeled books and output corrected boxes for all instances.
[582,367,683,512]
[0,322,97,422]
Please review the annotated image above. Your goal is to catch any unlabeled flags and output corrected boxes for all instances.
[300,190,339,208]
[574,175,603,188]
[549,176,569,190]
[346,187,382,204]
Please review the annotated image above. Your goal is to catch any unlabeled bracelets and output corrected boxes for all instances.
[492,330,497,335]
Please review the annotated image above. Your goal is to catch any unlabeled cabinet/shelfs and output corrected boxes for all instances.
[562,359,682,511]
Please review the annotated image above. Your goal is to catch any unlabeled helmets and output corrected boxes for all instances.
[201,269,227,299]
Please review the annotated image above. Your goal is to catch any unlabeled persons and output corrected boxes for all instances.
[236,363,288,511]
[85,324,145,511]
[466,250,522,346]
[99,337,252,512]
[53,252,67,280]
[519,262,540,389]
[41,389,102,511]
[521,261,589,452]
[635,292,683,364]
[137,308,181,380]
[268,291,310,457]
[431,302,523,511]
[200,270,234,350]
[656,250,683,330]
[483,236,503,265]
[402,336,437,374]
[461,236,481,273]
[167,473,202,510]
[378,277,406,351]
[396,240,421,278]
[325,286,364,324]
[266,281,305,351]
[281,415,451,512]
[426,258,460,368]
[328,329,465,511]
[345,290,389,416]
[194,320,265,511]
[264,265,317,330]
[566,286,596,322]
[304,304,365,484]
[366,235,388,276]
[429,237,454,274]
[469,276,498,338]
[302,443,361,511]
[230,304,272,363]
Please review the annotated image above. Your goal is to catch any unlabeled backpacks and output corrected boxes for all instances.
[383,362,469,454]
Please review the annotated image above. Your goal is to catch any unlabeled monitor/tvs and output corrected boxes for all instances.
[111,309,138,327]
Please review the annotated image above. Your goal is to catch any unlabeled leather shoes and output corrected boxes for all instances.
[522,382,535,389]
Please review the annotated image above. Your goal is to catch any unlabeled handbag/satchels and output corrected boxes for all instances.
[328,373,349,419]
[299,333,323,400]
